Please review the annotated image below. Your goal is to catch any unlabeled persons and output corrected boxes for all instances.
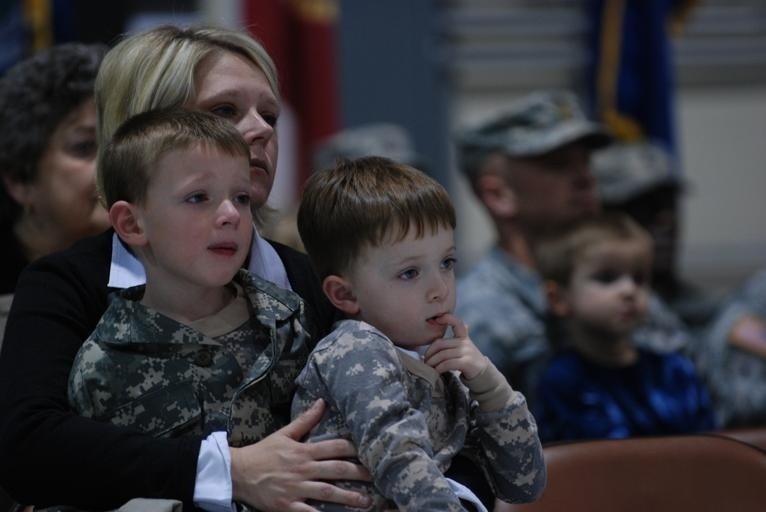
[2,27,546,512]
[435,89,764,446]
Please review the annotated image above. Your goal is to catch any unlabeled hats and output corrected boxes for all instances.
[590,142,695,206]
[313,119,428,178]
[449,87,611,189]
